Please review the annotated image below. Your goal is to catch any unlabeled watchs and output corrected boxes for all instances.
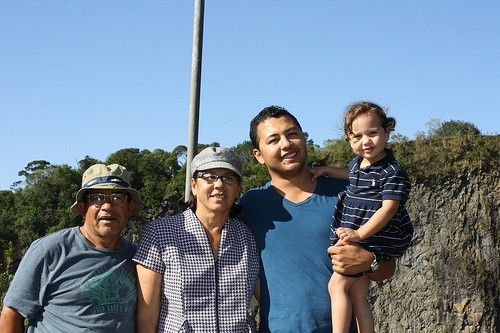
[367,252,381,273]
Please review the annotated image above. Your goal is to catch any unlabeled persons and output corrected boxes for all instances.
[0,163,145,333]
[132,147,260,333]
[236,104,396,333]
[309,101,413,333]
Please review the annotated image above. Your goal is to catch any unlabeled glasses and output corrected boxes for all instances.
[194,172,240,184]
[86,194,131,205]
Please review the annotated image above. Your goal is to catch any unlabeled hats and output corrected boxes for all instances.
[70,164,145,217]
[191,147,243,179]
